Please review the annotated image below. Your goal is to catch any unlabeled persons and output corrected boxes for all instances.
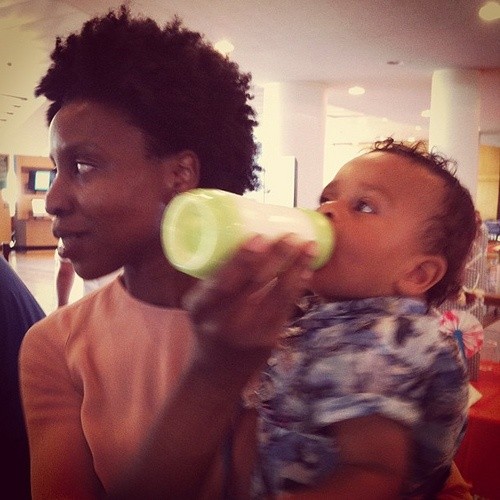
[56,237,124,308]
[248,136,482,500]
[0,254,47,500]
[19,3,474,500]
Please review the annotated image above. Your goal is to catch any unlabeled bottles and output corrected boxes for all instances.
[162,190,335,280]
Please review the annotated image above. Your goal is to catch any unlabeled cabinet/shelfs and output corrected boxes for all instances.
[16,219,58,247]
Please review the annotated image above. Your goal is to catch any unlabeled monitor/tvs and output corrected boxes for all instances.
[28,170,56,192]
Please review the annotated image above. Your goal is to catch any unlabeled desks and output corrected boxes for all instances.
[438,360,500,500]
